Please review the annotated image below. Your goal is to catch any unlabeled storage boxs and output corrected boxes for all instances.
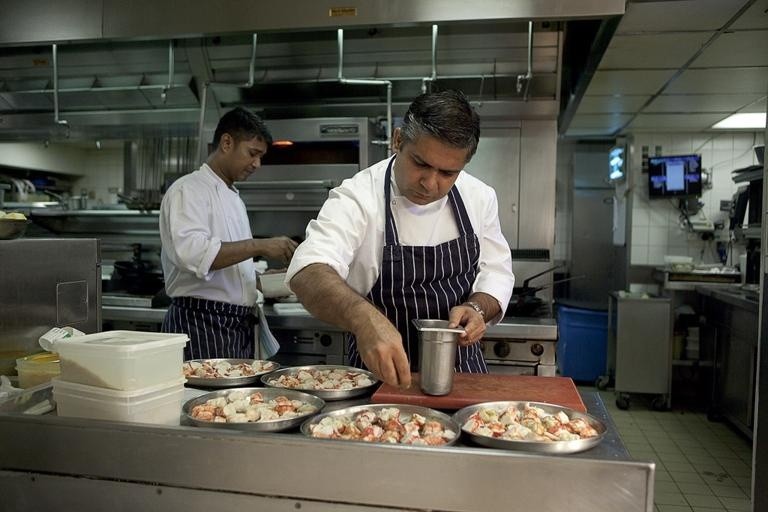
[0,330,190,428]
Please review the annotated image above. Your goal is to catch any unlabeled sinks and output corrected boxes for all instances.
[273,302,323,327]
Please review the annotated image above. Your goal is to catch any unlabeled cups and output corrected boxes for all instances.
[412,319,465,395]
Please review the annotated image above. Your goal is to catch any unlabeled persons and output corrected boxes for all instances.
[283,86,517,387]
[158,103,300,367]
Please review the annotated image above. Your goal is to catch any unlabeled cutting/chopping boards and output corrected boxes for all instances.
[371,373,587,415]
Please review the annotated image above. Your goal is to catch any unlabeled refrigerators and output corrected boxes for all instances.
[569,151,615,306]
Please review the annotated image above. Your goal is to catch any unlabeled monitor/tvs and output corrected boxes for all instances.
[648,155,701,199]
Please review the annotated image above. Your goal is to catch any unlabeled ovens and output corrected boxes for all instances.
[204,117,387,213]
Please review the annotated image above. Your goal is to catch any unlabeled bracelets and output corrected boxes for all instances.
[463,300,483,317]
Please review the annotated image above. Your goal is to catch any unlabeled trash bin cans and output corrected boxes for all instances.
[557,298,615,385]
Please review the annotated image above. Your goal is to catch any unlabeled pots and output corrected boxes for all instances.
[45,190,87,210]
[113,260,154,274]
[117,193,160,210]
[509,286,547,303]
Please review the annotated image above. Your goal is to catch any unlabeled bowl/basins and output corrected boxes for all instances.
[259,273,290,298]
[1,71,191,91]
[664,255,696,272]
[0,218,32,239]
[686,327,699,359]
[754,145,765,164]
